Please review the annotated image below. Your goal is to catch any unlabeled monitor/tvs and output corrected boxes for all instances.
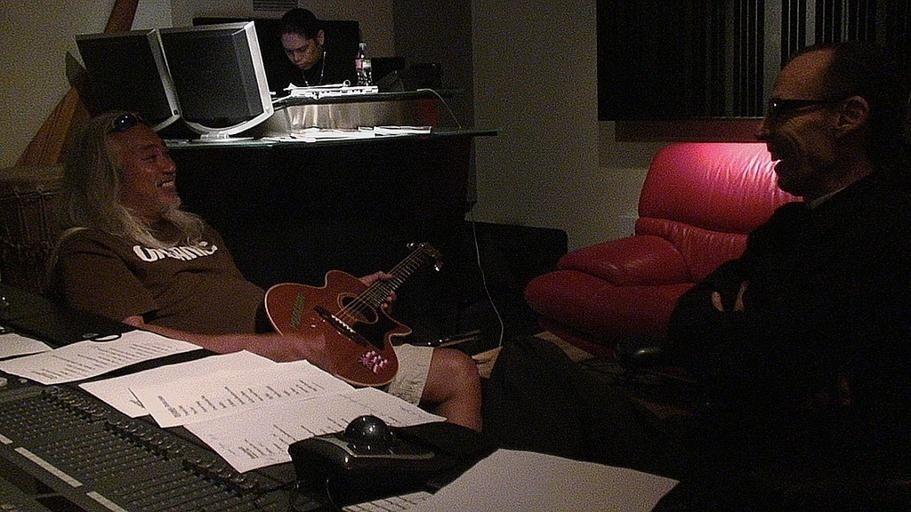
[73,28,180,135]
[159,20,275,143]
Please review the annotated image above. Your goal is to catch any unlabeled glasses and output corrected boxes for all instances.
[110,108,157,132]
[765,97,842,117]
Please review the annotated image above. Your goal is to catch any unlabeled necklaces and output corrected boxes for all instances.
[299,50,326,86]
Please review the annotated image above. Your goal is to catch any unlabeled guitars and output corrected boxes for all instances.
[264,240,442,386]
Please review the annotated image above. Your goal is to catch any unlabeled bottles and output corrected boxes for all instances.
[356,41,373,85]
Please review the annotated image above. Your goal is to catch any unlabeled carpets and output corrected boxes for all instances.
[466,325,697,419]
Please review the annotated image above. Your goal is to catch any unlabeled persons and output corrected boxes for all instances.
[269,7,359,94]
[580,38,911,512]
[38,110,484,436]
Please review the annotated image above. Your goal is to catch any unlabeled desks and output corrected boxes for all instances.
[158,122,499,340]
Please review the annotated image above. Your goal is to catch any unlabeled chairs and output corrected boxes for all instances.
[348,53,407,93]
[520,137,804,363]
[42,223,96,304]
